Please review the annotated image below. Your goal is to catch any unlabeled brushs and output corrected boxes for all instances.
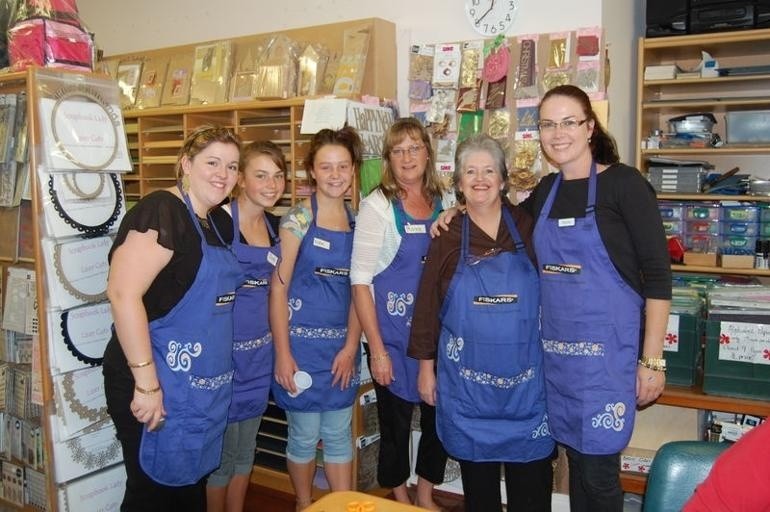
[701,167,740,191]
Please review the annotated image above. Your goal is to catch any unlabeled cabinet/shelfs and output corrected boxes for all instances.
[616,28,770,498]
[2,70,59,512]
[123,98,374,202]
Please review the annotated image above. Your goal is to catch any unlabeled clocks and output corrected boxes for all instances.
[466,0,517,36]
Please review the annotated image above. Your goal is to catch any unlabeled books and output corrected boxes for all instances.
[647,164,707,194]
[708,276,770,311]
[645,63,770,80]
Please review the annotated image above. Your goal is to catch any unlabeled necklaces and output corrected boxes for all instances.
[195,214,210,229]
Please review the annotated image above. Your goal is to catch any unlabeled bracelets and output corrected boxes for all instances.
[637,356,667,373]
[368,353,389,361]
[127,356,154,368]
[134,385,160,395]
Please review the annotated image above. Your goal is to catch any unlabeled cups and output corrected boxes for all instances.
[286,371,312,398]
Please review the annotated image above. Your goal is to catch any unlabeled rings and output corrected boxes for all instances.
[438,209,446,217]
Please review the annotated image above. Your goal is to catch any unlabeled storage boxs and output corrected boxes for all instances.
[701,311,769,394]
[657,198,770,259]
[724,111,770,145]
[664,314,698,389]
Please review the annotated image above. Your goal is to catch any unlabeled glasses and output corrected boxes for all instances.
[538,117,588,131]
[389,144,426,157]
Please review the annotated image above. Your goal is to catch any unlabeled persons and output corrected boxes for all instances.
[267,125,365,512]
[100,121,247,511]
[348,117,453,512]
[202,137,289,512]
[429,84,675,512]
[405,132,561,512]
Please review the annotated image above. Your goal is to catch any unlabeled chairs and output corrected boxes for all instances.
[639,440,731,512]
